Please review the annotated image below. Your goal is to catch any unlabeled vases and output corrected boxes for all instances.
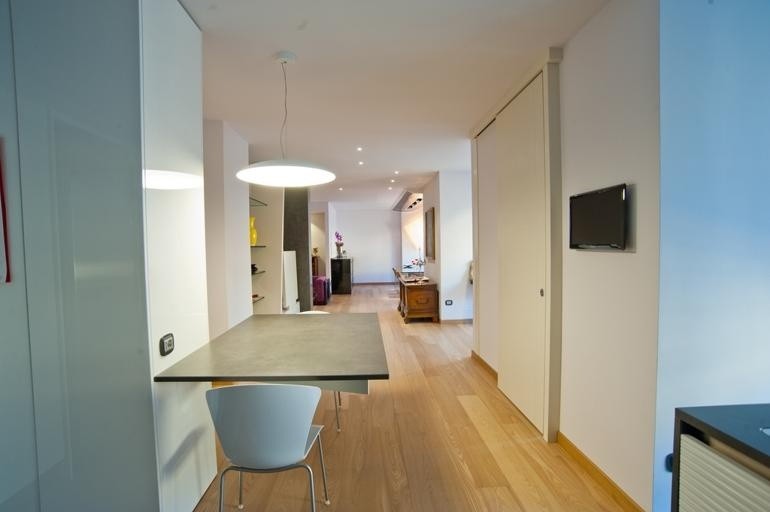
[336,242,344,258]
[250,217,258,246]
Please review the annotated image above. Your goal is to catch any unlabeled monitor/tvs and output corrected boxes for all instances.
[569,181,627,252]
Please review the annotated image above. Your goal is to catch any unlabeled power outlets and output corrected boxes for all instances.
[160,333,174,356]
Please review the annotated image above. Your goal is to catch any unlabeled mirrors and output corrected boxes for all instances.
[425,206,435,261]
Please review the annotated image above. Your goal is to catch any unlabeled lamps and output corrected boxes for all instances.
[236,61,336,188]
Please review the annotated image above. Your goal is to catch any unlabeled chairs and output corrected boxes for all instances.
[205,385,330,512]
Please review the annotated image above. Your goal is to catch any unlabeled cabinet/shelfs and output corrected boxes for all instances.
[392,265,440,324]
[331,256,352,294]
[248,138,284,315]
[671,403,770,512]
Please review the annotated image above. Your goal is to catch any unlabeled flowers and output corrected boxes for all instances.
[335,232,342,242]
[412,258,424,267]
